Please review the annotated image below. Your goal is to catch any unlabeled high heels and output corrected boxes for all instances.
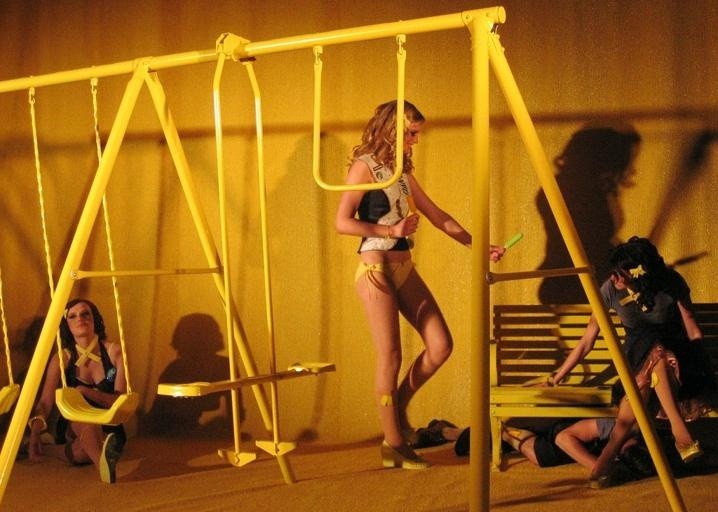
[381,439,431,470]
[97,430,124,486]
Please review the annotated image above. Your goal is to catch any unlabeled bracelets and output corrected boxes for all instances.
[26,415,48,434]
[383,226,391,243]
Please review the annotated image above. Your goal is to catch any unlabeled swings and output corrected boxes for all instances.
[28,80,139,426]
[0,280,21,416]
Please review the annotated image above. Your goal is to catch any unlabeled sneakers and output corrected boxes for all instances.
[416,419,458,447]
[455,426,470,457]
[401,428,428,449]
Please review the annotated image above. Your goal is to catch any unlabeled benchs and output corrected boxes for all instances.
[489,297,718,472]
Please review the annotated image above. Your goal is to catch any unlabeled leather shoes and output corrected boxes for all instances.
[674,440,703,464]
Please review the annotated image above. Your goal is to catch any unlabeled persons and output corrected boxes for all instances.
[17,298,127,487]
[542,234,714,491]
[452,350,685,490]
[334,97,509,471]
